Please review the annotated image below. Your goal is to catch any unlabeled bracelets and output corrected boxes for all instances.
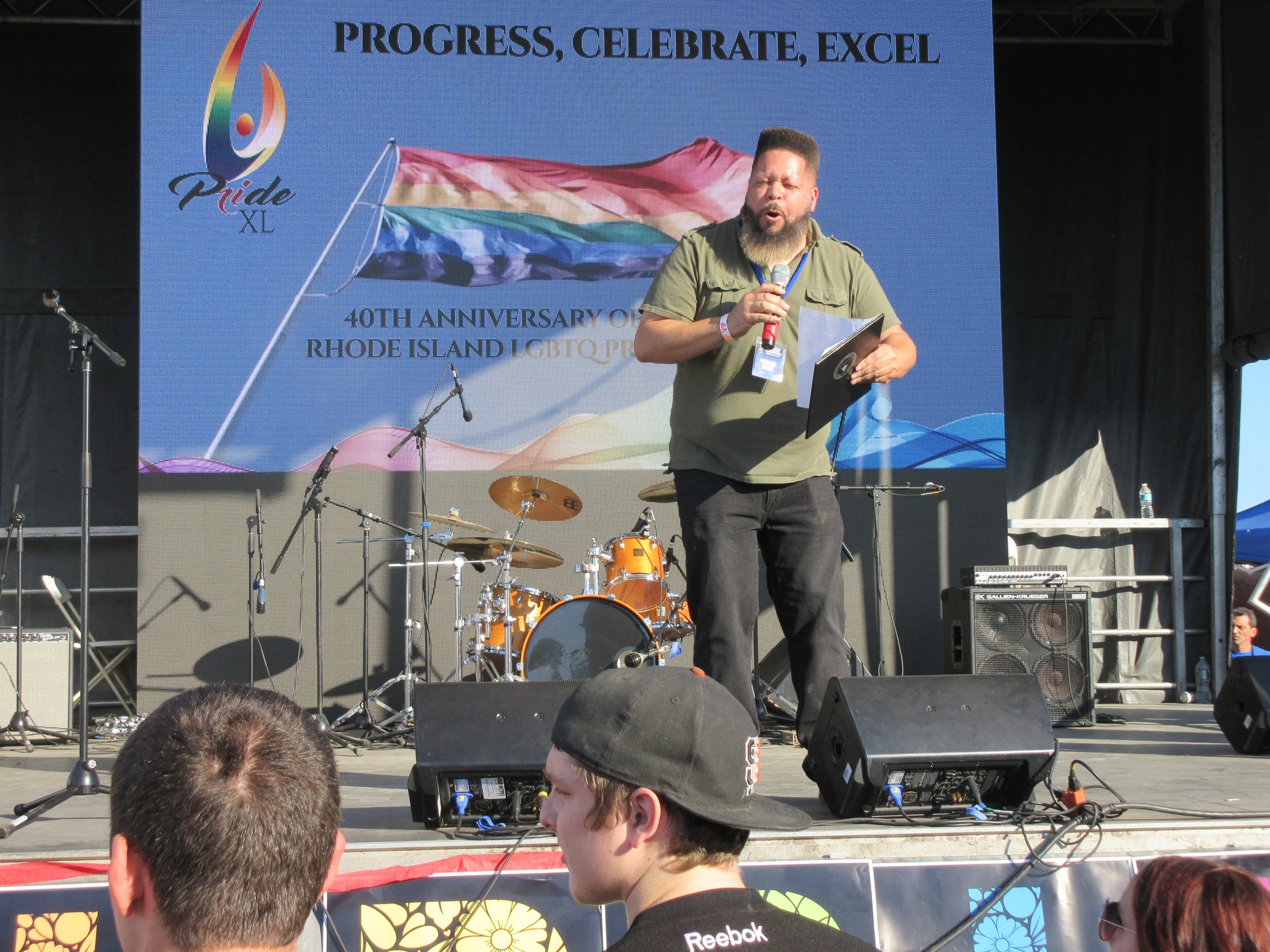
[719,313,736,344]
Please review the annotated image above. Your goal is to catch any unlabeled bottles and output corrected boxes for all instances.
[1195,657,1212,704]
[1179,691,1195,704]
[1139,483,1153,518]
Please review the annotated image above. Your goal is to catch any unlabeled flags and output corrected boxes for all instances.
[352,136,758,287]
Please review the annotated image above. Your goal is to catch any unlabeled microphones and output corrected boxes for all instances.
[625,643,670,670]
[662,538,677,579]
[764,262,789,351]
[449,361,475,422]
[305,445,338,494]
[256,577,265,616]
[465,555,487,574]
[630,507,651,534]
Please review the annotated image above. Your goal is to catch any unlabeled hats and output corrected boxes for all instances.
[550,665,813,831]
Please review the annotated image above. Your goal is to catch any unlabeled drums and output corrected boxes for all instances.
[601,532,665,614]
[639,592,695,641]
[469,581,561,672]
[519,594,664,681]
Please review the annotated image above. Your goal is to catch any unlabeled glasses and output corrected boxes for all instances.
[1099,902,1138,942]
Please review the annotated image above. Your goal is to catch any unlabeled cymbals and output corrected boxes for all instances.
[404,512,497,534]
[638,480,677,502]
[486,473,584,523]
[446,535,564,569]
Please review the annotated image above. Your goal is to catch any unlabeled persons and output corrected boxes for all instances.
[1230,606,1270,664]
[1098,857,1270,952]
[106,683,348,952]
[632,127,917,752]
[539,666,886,952]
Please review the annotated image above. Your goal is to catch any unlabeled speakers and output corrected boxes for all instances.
[803,669,1060,815]
[942,587,1098,729]
[407,684,589,827]
[1214,655,1270,756]
